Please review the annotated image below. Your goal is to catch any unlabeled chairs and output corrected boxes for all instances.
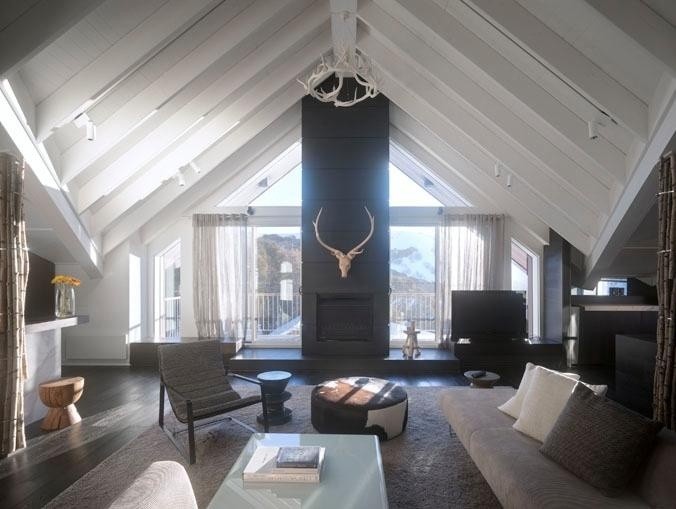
[156,338,270,466]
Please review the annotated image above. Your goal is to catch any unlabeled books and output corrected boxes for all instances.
[240,443,326,485]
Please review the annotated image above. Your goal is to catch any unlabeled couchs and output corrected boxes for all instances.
[438,386,676,508]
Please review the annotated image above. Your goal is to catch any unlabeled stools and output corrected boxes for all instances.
[256,370,409,442]
[38,376,85,431]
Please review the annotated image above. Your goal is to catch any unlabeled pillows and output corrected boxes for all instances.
[496,361,668,500]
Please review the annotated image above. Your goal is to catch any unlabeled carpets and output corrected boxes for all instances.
[42,381,515,508]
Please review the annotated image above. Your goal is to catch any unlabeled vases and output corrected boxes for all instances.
[53,282,76,318]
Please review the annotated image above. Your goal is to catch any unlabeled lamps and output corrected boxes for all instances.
[494,161,514,188]
[72,113,98,143]
[588,113,615,140]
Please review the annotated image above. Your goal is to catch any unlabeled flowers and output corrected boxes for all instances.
[50,274,81,316]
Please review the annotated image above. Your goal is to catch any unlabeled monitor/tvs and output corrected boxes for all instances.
[451,290,528,342]
[24,250,55,324]
